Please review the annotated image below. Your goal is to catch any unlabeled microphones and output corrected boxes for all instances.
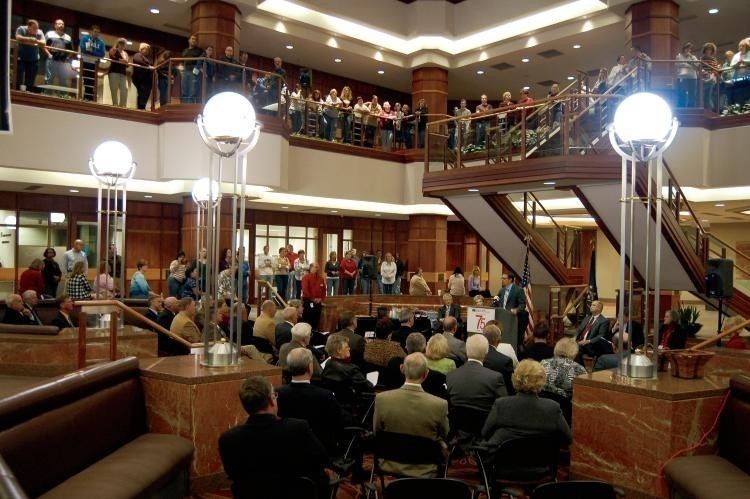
[493,294,501,305]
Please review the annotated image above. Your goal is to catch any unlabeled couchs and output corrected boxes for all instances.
[663,375,746,494]
[1,356,195,495]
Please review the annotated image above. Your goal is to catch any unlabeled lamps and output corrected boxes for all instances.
[88,140,138,329]
[605,92,681,378]
[196,92,265,368]
[191,177,222,299]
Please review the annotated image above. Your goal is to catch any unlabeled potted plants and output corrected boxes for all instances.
[678,306,702,337]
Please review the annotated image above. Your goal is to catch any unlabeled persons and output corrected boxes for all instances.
[481,358,571,499]
[169,298,211,344]
[342,251,356,295]
[180,269,207,301]
[360,251,377,294]
[627,45,651,94]
[302,264,326,331]
[425,334,457,372]
[698,42,720,111]
[380,102,397,150]
[274,307,315,349]
[326,251,340,297]
[675,42,700,108]
[233,52,252,102]
[332,311,368,363]
[393,253,403,295]
[155,297,181,336]
[729,38,750,114]
[364,94,383,148]
[363,319,407,375]
[473,295,485,308]
[720,51,737,116]
[387,332,446,389]
[415,99,429,148]
[437,294,462,328]
[140,295,163,332]
[201,46,216,103]
[51,295,82,328]
[353,97,370,147]
[609,307,644,354]
[61,239,88,295]
[132,42,153,112]
[289,83,304,135]
[443,266,466,295]
[16,19,46,93]
[443,335,507,440]
[236,249,251,306]
[64,261,93,300]
[349,248,361,294]
[294,251,310,299]
[103,244,125,299]
[94,262,124,301]
[167,252,189,297]
[439,317,467,358]
[593,331,634,370]
[108,38,129,108]
[517,89,538,130]
[80,26,105,102]
[468,266,492,298]
[275,249,291,304]
[400,105,416,150]
[253,70,271,115]
[593,68,609,124]
[610,55,628,95]
[475,95,493,150]
[285,244,301,301]
[542,338,590,402]
[654,310,687,350]
[218,47,241,91]
[547,85,563,125]
[373,352,449,481]
[482,319,520,368]
[18,257,44,299]
[322,89,343,143]
[218,376,328,499]
[270,57,287,116]
[197,300,236,352]
[580,77,594,115]
[375,250,386,294]
[258,247,274,301]
[495,272,526,315]
[129,259,162,299]
[575,301,612,368]
[518,322,553,360]
[339,87,354,143]
[38,248,61,299]
[157,49,177,106]
[409,268,432,297]
[393,102,403,150]
[305,90,323,137]
[252,300,280,348]
[381,253,397,295]
[320,334,372,436]
[450,107,461,150]
[455,99,472,152]
[42,19,74,98]
[280,324,325,384]
[181,35,207,104]
[372,307,397,334]
[218,246,239,275]
[191,246,213,295]
[393,310,426,350]
[21,291,43,326]
[497,92,516,149]
[4,294,38,325]
[213,300,230,328]
[270,348,372,484]
[478,325,517,395]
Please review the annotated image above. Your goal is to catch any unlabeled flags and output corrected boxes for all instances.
[586,238,598,317]
[522,235,539,339]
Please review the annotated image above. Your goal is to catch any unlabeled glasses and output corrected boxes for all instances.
[502,278,508,280]
[272,392,278,399]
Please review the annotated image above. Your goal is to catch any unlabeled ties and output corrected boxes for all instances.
[503,289,507,309]
[446,306,449,316]
[68,316,75,328]
[579,317,594,341]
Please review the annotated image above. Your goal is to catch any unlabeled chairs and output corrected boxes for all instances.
[470,429,559,498]
[225,470,342,499]
[364,430,459,498]
[365,476,480,499]
[502,480,625,498]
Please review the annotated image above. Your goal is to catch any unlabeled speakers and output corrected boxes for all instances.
[299,68,312,88]
[358,255,379,281]
[705,259,733,299]
